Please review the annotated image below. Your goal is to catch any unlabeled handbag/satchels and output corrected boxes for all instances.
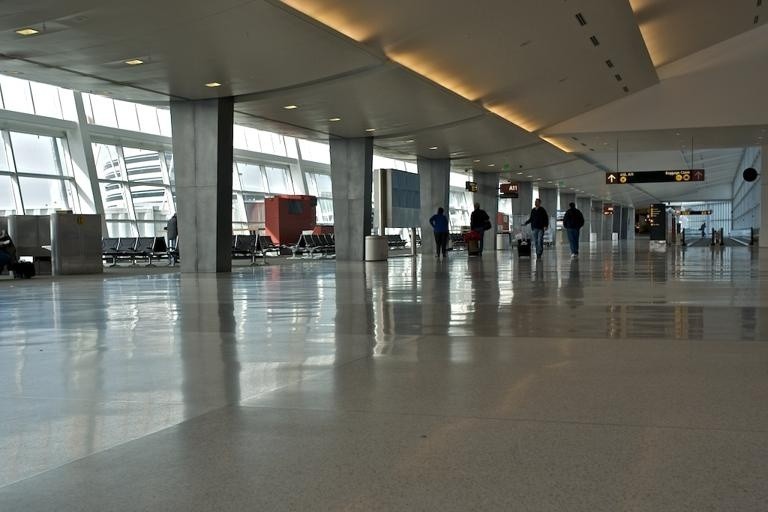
[17,262,36,275]
[479,216,491,230]
[466,231,480,240]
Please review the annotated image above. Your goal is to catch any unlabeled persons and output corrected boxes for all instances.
[563,203,584,257]
[521,199,549,258]
[470,203,490,256]
[429,207,448,257]
[168,213,178,247]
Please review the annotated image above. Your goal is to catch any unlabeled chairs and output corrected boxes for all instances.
[231,232,335,263]
[101,235,179,269]
[387,233,407,250]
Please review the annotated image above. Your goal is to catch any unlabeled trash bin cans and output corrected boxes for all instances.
[365,235,388,261]
[611,231,619,241]
[496,233,510,250]
[590,232,597,242]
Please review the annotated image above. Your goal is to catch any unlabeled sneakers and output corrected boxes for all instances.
[570,253,578,258]
[537,253,541,258]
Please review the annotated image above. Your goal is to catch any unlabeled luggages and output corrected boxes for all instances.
[517,224,532,257]
[467,240,481,255]
